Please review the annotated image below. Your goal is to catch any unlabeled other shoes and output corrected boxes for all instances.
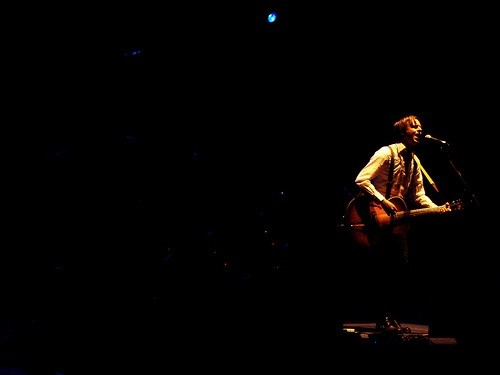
[392,320,411,333]
[376,319,403,333]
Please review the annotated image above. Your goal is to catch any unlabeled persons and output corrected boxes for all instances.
[353,113,465,341]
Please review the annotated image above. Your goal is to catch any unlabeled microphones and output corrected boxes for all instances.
[424,134,448,145]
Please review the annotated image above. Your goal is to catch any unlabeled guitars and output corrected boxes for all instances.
[342,193,464,247]
